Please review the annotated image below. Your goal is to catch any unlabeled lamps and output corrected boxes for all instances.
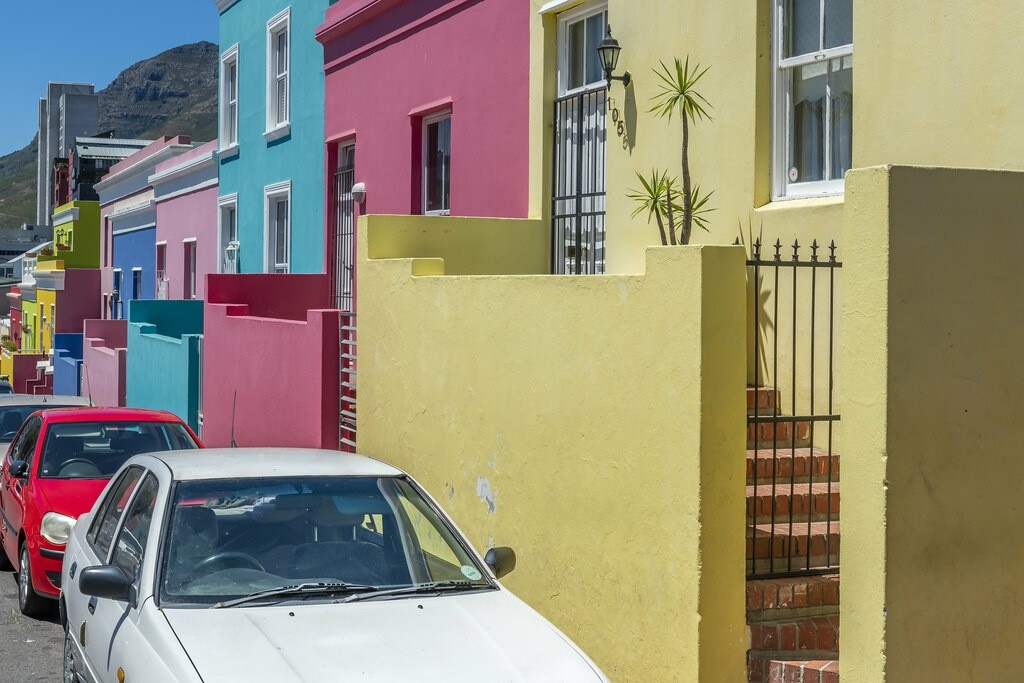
[226,241,240,263]
[113,286,123,304]
[352,182,366,203]
[597,24,631,91]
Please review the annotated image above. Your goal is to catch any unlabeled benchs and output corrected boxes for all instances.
[217,499,315,569]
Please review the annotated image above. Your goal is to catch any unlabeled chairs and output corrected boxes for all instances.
[44,437,84,476]
[166,507,225,591]
[109,434,156,472]
[0,412,23,441]
[291,493,392,585]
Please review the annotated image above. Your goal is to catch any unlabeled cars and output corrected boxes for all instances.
[59,385,613,683]
[0,383,241,617]
